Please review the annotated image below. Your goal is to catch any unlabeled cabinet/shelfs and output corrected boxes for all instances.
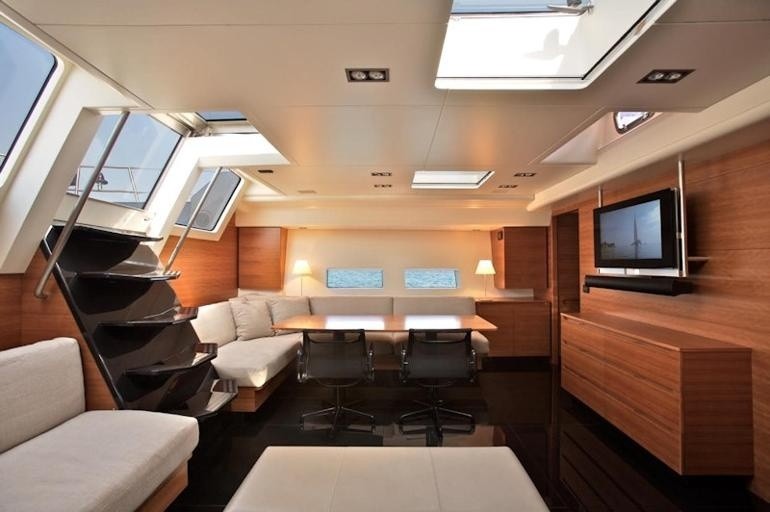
[489,226,549,290]
[476,297,551,370]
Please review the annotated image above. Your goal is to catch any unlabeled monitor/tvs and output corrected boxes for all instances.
[592,188,677,268]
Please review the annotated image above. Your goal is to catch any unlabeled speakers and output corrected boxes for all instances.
[584,275,693,296]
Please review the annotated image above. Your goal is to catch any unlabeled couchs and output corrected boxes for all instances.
[189,293,489,434]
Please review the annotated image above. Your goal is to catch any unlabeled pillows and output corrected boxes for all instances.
[307,295,393,315]
[266,295,311,336]
[238,288,286,297]
[393,295,476,315]
[228,295,274,341]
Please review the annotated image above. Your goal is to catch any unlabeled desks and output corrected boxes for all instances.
[559,311,752,504]
[270,315,498,432]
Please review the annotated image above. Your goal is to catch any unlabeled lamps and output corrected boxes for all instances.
[293,260,312,296]
[474,260,497,297]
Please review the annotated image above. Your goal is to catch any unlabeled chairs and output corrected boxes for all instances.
[295,330,375,440]
[398,329,475,447]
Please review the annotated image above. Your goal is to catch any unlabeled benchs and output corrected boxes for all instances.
[0,337,201,512]
